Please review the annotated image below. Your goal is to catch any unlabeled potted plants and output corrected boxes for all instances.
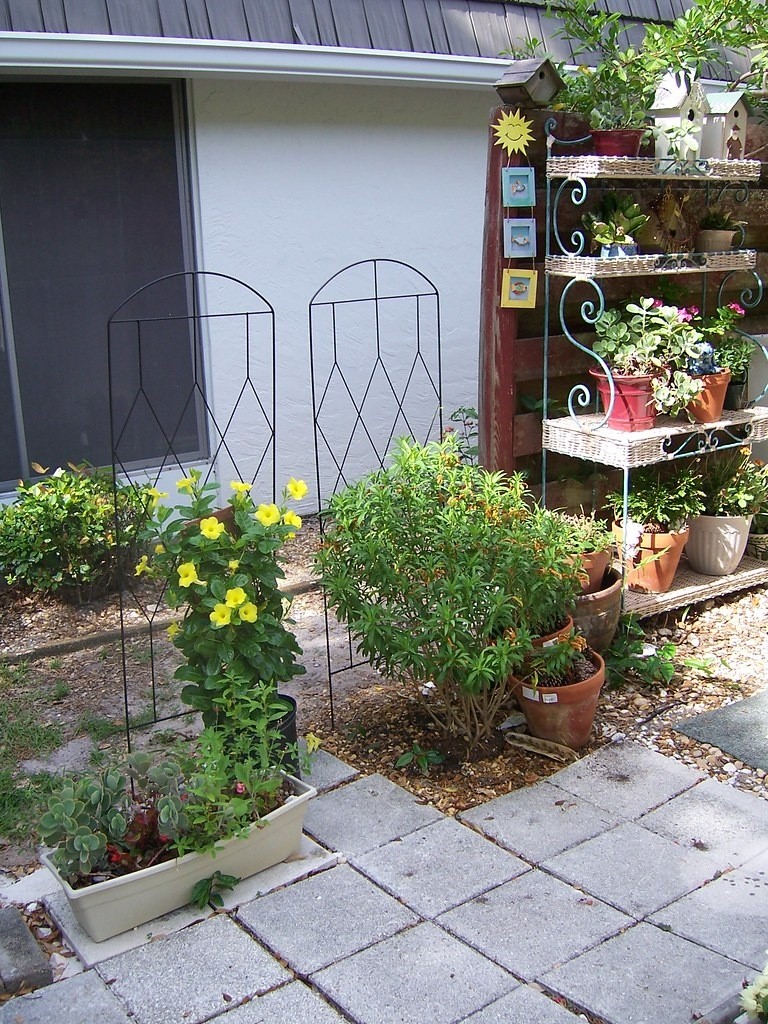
[588,293,693,433]
[579,190,741,265]
[40,670,319,944]
[585,93,699,161]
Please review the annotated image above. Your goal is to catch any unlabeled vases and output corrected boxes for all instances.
[671,369,732,424]
[200,695,302,786]
[467,509,755,754]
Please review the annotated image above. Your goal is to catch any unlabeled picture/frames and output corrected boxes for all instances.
[500,166,539,309]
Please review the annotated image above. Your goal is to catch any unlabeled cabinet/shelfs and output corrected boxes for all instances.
[541,116,768,636]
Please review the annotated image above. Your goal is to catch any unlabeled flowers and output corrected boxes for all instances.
[669,438,768,500]
[136,458,336,654]
[651,290,749,378]
